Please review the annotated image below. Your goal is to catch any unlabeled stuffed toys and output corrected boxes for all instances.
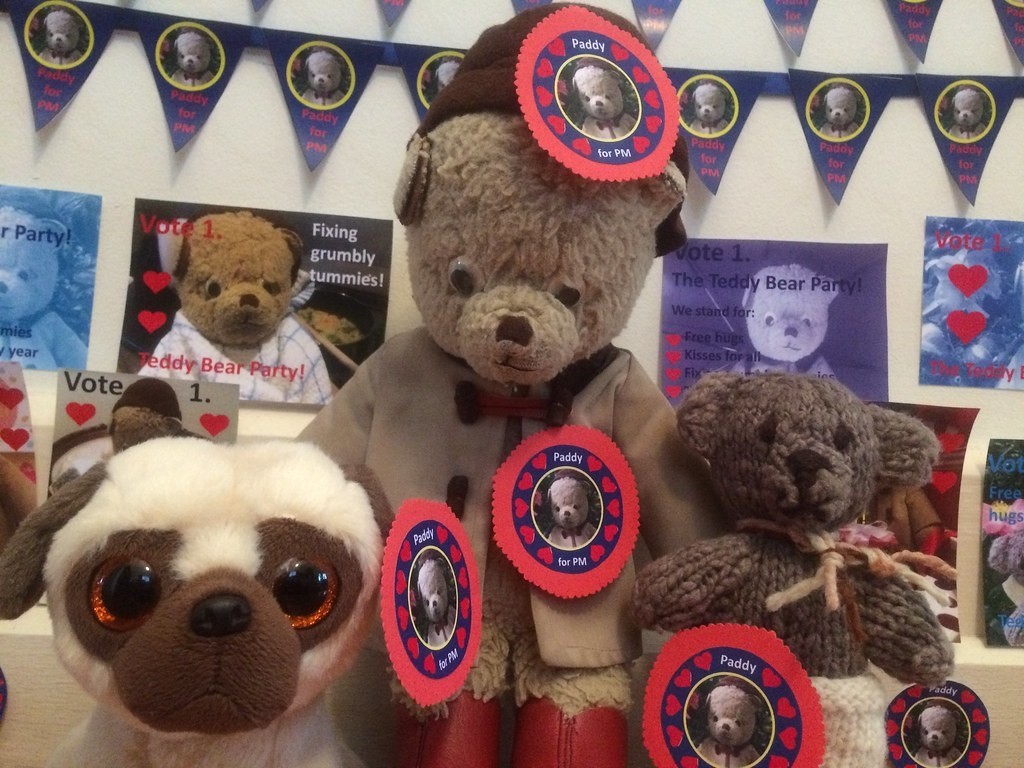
[988,530,1024,647]
[0,435,395,768]
[730,266,839,378]
[416,558,461,647]
[305,45,347,105]
[290,1,736,768]
[166,30,219,86]
[635,372,947,768]
[913,707,965,768]
[569,65,638,140]
[546,478,596,550]
[695,686,763,768]
[137,214,333,406]
[688,78,728,130]
[0,210,89,368]
[817,85,862,135]
[434,61,461,92]
[948,89,990,138]
[37,12,85,62]
